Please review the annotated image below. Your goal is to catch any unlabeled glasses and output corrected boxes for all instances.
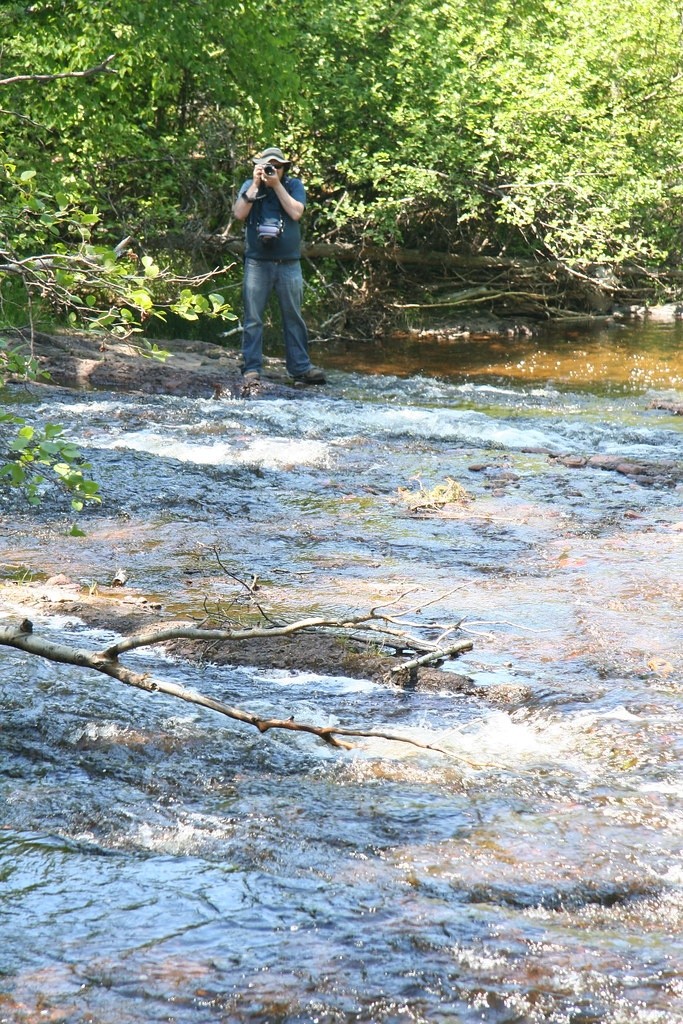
[273,164,285,169]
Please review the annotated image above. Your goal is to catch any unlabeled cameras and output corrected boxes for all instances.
[264,163,274,175]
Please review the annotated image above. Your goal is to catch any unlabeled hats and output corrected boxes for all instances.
[252,147,292,173]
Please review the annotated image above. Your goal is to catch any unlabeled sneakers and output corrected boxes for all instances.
[299,367,327,383]
[243,368,260,380]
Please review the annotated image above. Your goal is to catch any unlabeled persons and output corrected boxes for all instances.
[233,147,325,384]
